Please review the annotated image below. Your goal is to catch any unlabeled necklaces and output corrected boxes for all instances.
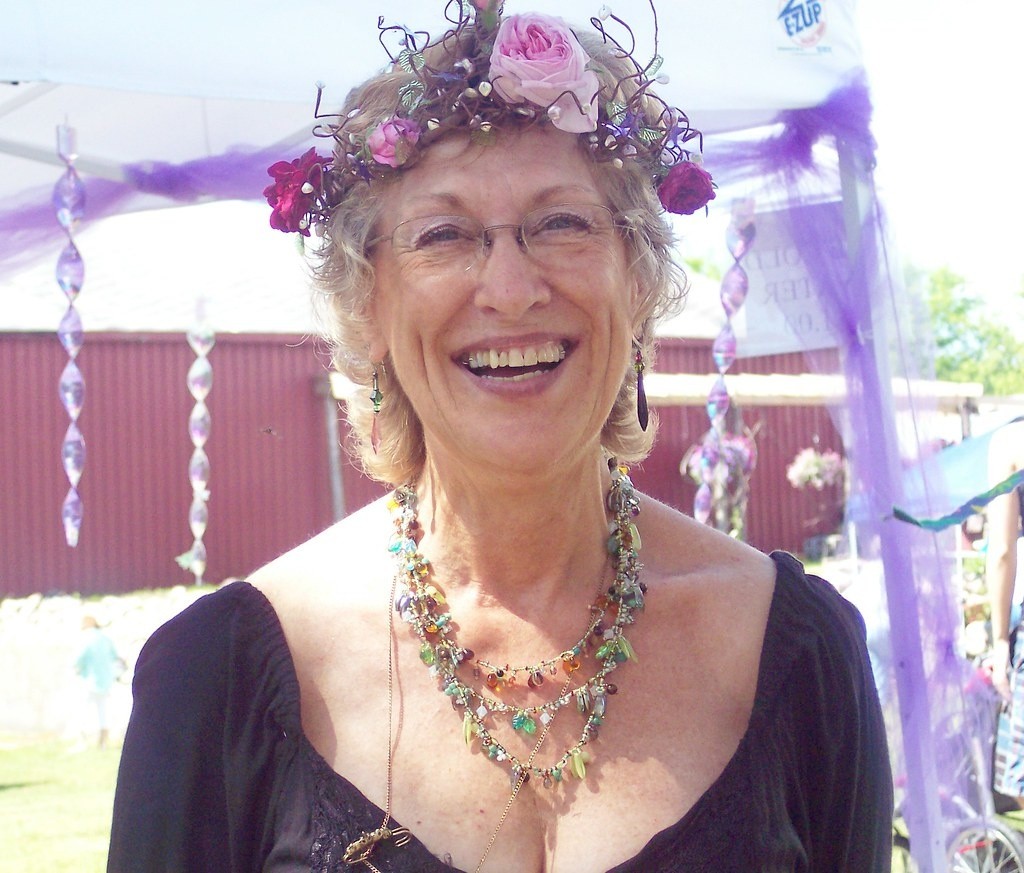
[343,455,646,873]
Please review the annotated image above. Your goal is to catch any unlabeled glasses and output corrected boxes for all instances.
[366,202,635,277]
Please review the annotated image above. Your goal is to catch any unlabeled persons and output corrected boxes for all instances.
[107,1,893,873]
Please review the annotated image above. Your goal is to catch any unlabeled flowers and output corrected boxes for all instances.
[261,145,333,238]
[365,114,419,171]
[659,160,715,217]
[485,12,599,136]
